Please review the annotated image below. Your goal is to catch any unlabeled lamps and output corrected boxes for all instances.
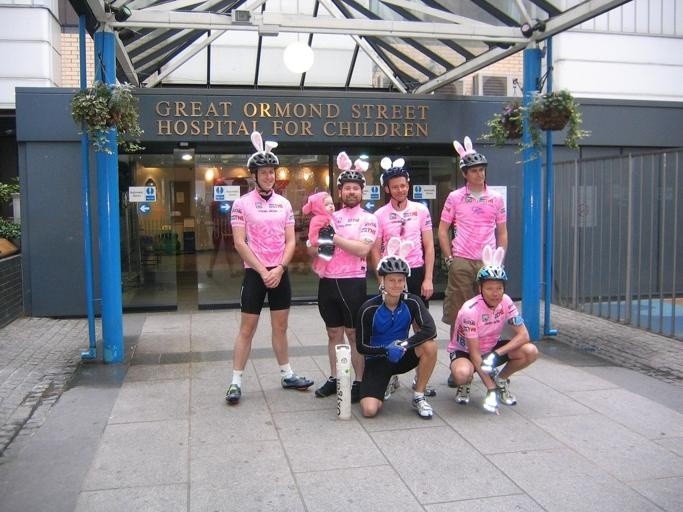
[521,18,546,38]
[283,0,313,74]
[105,3,131,22]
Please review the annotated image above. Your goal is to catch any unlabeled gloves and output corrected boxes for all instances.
[385,339,407,363]
[483,389,499,412]
[480,350,500,372]
[319,225,336,240]
[318,244,335,255]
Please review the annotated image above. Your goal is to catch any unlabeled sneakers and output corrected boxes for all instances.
[411,376,436,396]
[225,384,241,401]
[455,382,471,404]
[448,374,457,388]
[351,381,361,403]
[303,266,310,273]
[315,376,336,398]
[489,368,510,384]
[291,266,299,272]
[384,375,397,401]
[281,373,314,388]
[411,396,433,417]
[493,377,516,405]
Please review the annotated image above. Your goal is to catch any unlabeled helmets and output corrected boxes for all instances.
[378,257,409,276]
[460,153,488,167]
[476,266,508,284]
[383,167,408,186]
[249,153,279,173]
[338,171,365,189]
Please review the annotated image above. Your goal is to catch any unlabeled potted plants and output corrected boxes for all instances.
[70,55,145,155]
[476,78,524,150]
[514,66,591,165]
[0,176,21,225]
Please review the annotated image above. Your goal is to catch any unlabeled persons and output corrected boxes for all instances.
[225,131,314,402]
[447,245,539,416]
[437,136,508,387]
[302,191,339,280]
[206,181,236,279]
[316,152,378,403]
[371,157,436,400]
[355,255,438,418]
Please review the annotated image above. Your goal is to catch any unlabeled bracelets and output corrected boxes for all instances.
[279,263,286,272]
